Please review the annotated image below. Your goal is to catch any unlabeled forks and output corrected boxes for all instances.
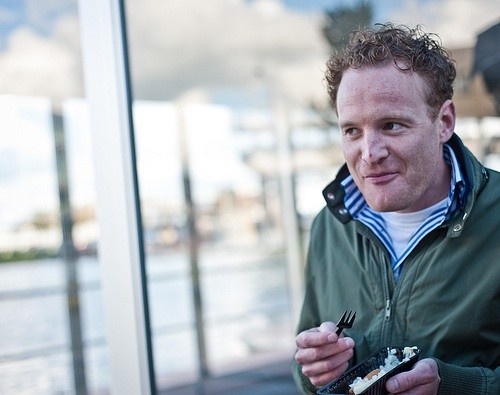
[334,310,357,336]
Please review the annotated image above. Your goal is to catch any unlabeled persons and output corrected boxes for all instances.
[291,23,499,395]
